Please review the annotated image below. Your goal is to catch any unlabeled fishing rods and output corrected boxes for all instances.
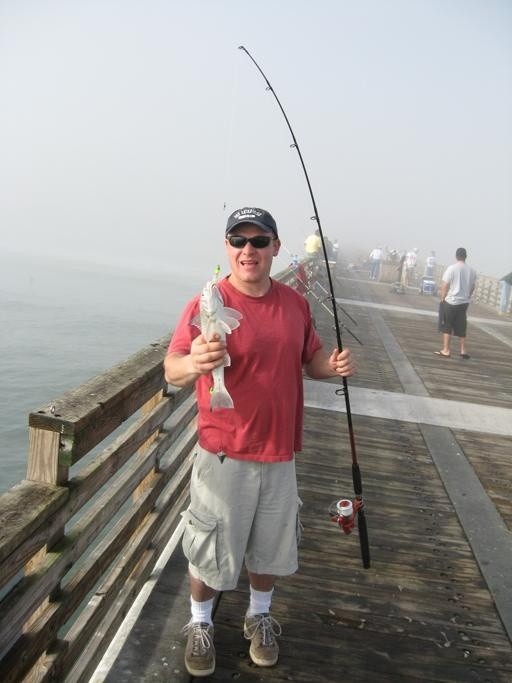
[283,245,358,327]
[275,253,364,347]
[238,45,371,570]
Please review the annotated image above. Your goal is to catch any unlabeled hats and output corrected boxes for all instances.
[225,207,278,239]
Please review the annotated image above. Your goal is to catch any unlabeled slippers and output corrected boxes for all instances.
[460,352,470,360]
[432,351,450,357]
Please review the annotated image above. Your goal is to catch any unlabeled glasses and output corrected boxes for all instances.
[226,234,275,248]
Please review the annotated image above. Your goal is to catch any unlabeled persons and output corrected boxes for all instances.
[162,204,356,679]
[304,228,439,289]
[432,247,479,359]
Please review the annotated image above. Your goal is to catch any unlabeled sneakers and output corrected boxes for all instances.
[183,622,217,678]
[243,612,282,668]
